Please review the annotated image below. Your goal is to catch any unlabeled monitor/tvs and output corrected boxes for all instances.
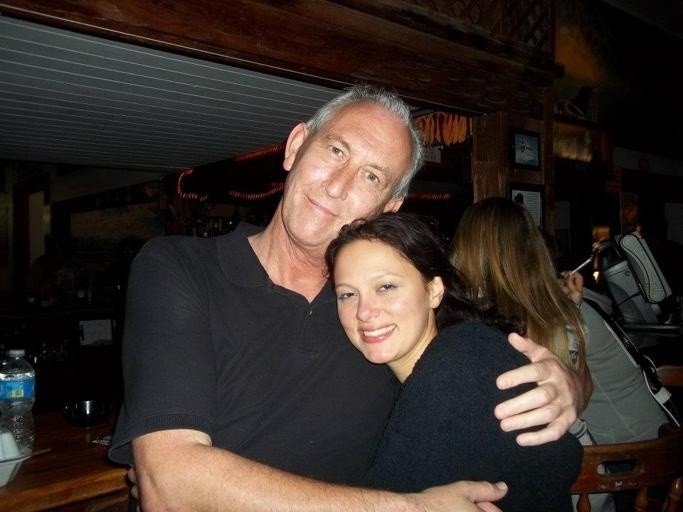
[77,317,114,347]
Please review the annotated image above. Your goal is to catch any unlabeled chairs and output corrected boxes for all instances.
[568,422,677,512]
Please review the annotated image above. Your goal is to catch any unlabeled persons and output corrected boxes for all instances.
[325,212,583,512]
[108,83,592,512]
[447,197,683,512]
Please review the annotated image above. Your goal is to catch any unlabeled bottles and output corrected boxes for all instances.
[0,350,35,458]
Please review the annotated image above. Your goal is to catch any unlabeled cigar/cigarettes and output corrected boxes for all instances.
[568,257,593,275]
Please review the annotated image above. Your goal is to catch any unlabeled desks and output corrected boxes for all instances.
[0,410,135,512]
